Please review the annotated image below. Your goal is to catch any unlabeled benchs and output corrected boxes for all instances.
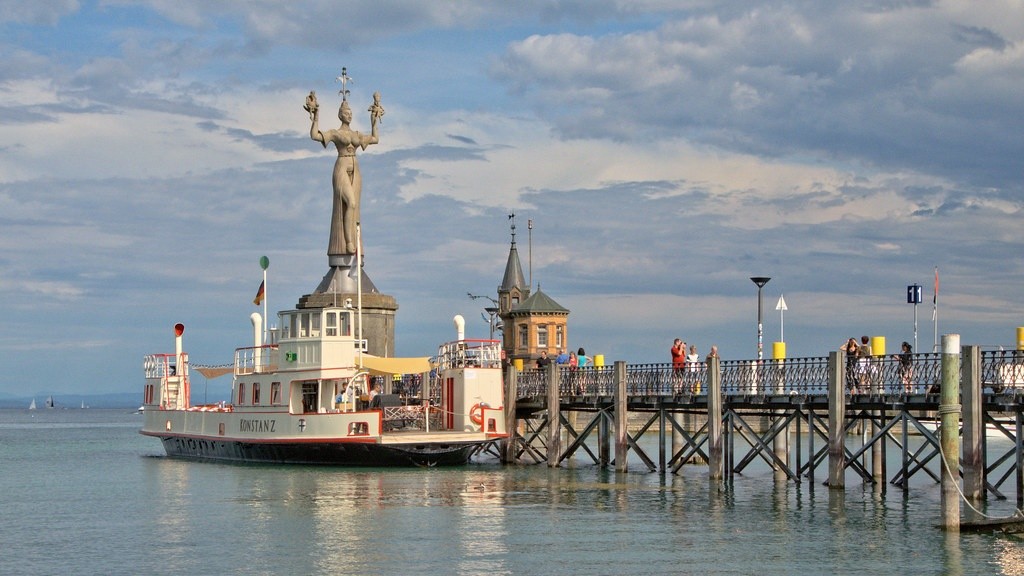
[382,405,422,428]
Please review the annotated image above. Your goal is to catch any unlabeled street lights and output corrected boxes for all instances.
[751,277,772,392]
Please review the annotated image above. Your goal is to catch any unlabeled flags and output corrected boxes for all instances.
[253,279,264,305]
[931,272,939,321]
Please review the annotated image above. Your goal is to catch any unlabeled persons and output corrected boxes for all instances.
[671,338,719,396]
[368,385,381,405]
[302,68,384,252]
[533,348,592,394]
[840,336,914,395]
[336,382,352,403]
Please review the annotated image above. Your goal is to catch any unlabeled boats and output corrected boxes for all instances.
[900,416,1023,438]
[138,222,510,467]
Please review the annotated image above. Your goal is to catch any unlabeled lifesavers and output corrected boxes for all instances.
[470,402,491,424]
